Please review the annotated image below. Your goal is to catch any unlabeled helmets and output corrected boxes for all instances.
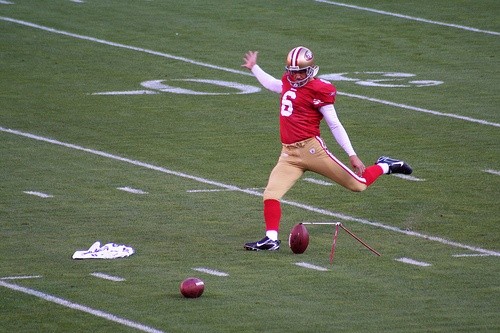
[285,46,319,87]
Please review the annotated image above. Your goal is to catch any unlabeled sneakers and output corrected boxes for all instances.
[244,236,281,252]
[375,156,412,175]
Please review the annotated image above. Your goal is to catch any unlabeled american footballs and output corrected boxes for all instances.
[180,277,205,298]
[289,223,310,254]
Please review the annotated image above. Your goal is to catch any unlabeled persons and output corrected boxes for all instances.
[240,46,414,251]
